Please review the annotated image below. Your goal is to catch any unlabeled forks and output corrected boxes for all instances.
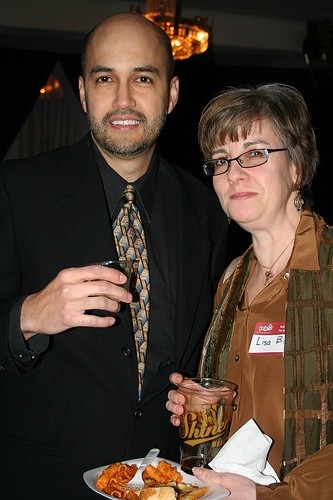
[122,448,160,491]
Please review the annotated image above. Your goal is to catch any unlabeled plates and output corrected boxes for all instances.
[83,458,231,500]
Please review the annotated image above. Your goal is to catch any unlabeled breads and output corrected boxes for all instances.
[125,486,177,500]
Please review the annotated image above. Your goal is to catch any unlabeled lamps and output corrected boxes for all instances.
[128,0,214,62]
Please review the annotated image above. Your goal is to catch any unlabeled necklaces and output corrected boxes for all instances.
[259,239,295,285]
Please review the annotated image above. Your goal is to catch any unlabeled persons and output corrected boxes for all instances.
[166,83,333,500]
[0,12,228,500]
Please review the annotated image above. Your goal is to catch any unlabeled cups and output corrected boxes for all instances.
[178,378,237,470]
[88,261,131,302]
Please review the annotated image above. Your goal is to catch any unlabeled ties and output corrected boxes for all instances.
[112,185,151,401]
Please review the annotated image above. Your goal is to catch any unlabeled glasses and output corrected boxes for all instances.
[202,148,288,176]
